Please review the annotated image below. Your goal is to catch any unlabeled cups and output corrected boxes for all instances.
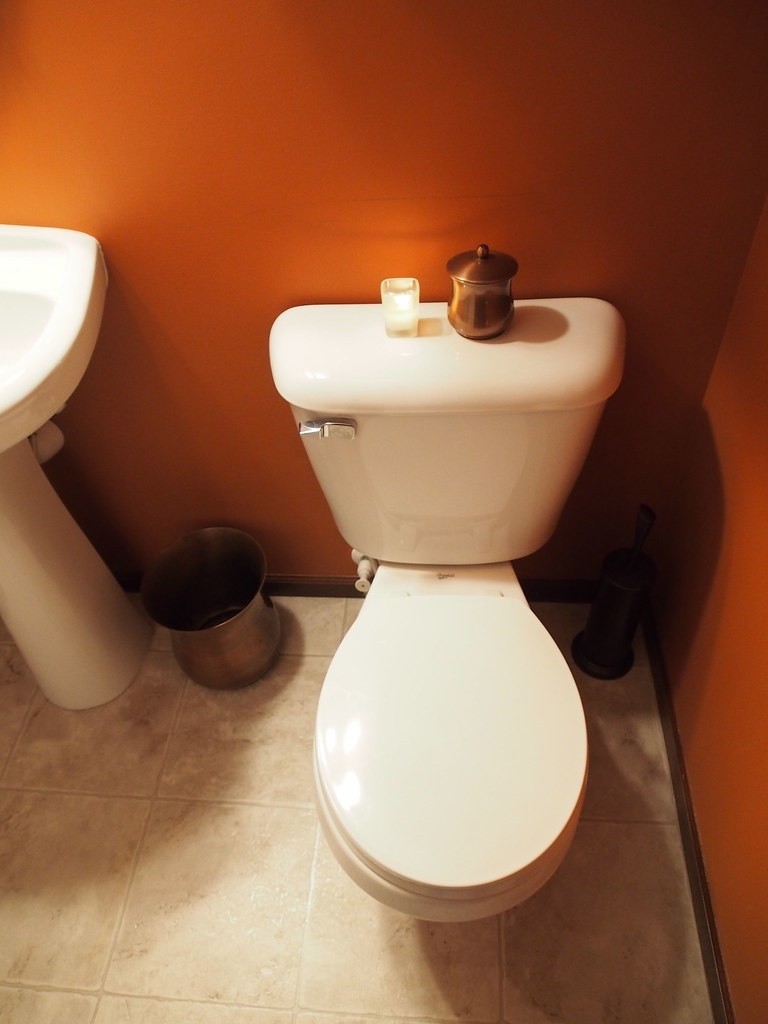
[379,277,420,337]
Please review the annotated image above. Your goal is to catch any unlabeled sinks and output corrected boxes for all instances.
[0,221,111,456]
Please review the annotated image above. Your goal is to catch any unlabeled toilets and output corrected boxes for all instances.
[268,295,628,924]
